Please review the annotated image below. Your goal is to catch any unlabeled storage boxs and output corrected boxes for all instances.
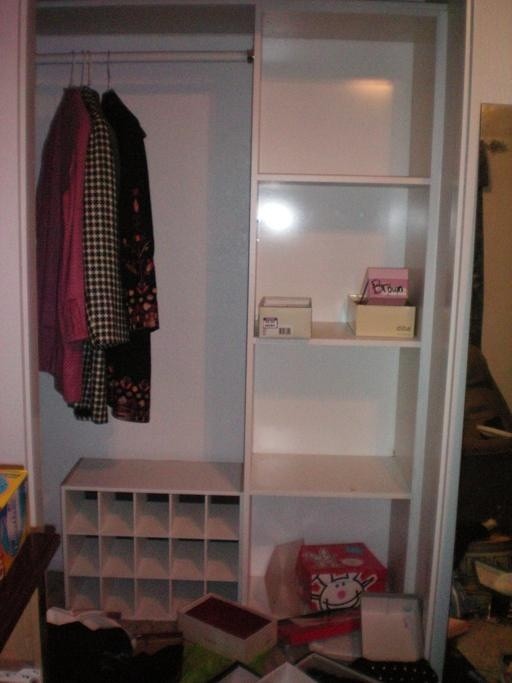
[346,295,415,338]
[0,459,29,582]
[360,267,409,305]
[257,295,313,339]
[175,541,425,683]
[0,0,475,683]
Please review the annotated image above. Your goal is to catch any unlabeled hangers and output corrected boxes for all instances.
[69,50,112,92]
